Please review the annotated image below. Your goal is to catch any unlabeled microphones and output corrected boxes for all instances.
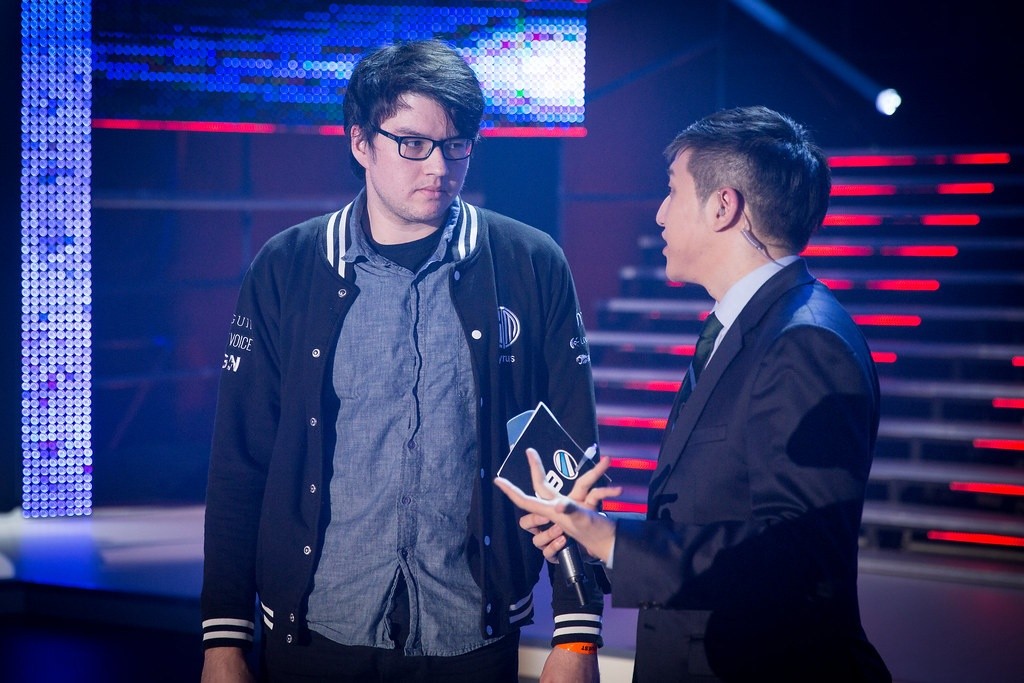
[508,411,589,606]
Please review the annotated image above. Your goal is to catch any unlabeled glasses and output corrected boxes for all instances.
[374,128,475,161]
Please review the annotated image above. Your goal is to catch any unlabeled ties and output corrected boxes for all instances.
[659,312,725,462]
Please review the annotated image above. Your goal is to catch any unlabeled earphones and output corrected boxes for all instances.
[719,207,725,217]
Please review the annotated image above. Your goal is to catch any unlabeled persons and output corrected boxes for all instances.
[493,104,895,683]
[198,40,605,683]
